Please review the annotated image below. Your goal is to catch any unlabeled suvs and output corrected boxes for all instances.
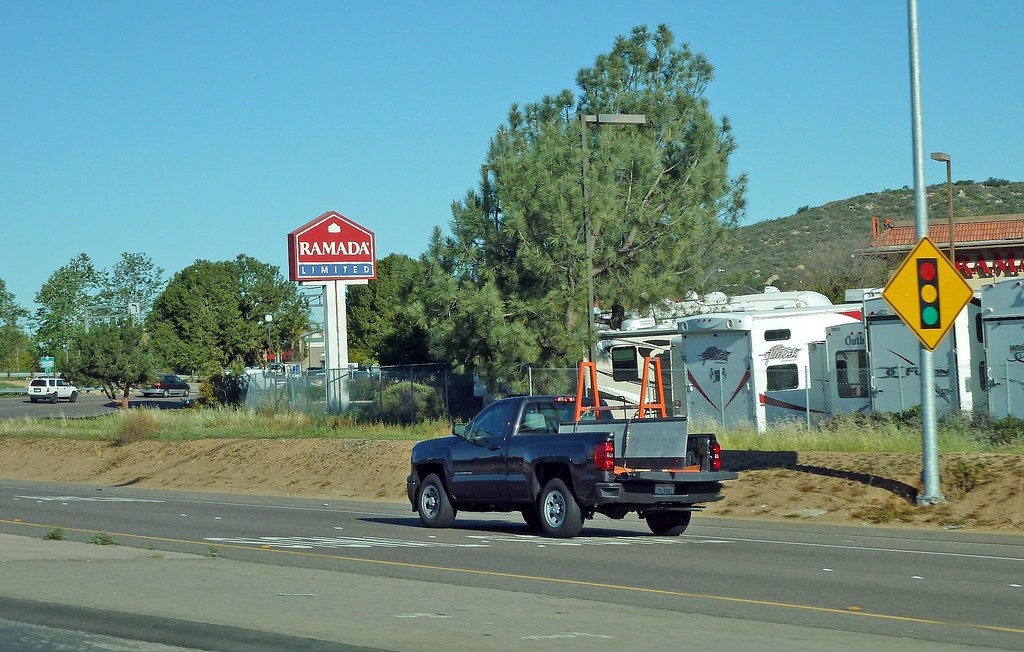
[28,377,78,404]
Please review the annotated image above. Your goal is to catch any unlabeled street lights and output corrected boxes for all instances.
[265,314,273,373]
[931,152,955,265]
[580,114,648,359]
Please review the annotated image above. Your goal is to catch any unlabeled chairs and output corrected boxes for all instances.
[524,413,545,429]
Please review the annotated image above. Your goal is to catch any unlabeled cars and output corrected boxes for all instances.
[141,372,190,398]
[271,362,379,385]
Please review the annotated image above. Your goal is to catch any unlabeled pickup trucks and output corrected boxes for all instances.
[406,394,739,539]
